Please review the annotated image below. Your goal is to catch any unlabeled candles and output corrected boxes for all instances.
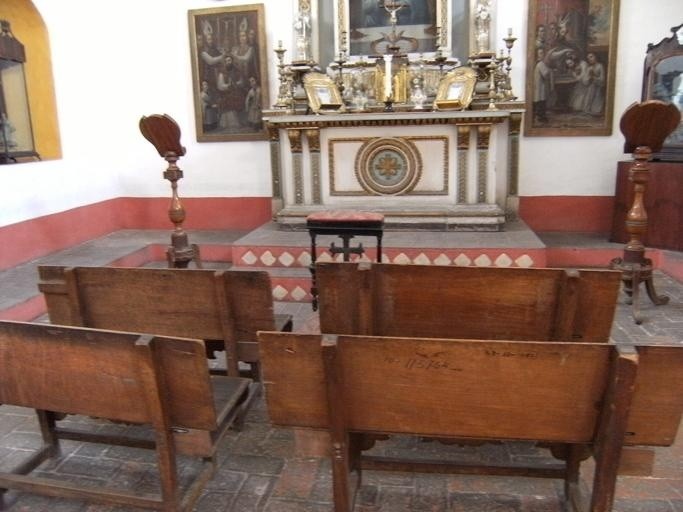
[278,39,283,47]
[507,27,512,36]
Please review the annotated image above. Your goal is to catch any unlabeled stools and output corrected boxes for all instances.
[307,210,385,312]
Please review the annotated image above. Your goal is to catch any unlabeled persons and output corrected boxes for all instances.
[195,29,263,134]
[532,22,604,126]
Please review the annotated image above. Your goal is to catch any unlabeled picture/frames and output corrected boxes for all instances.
[523,0,620,137]
[301,1,479,116]
[187,3,271,143]
